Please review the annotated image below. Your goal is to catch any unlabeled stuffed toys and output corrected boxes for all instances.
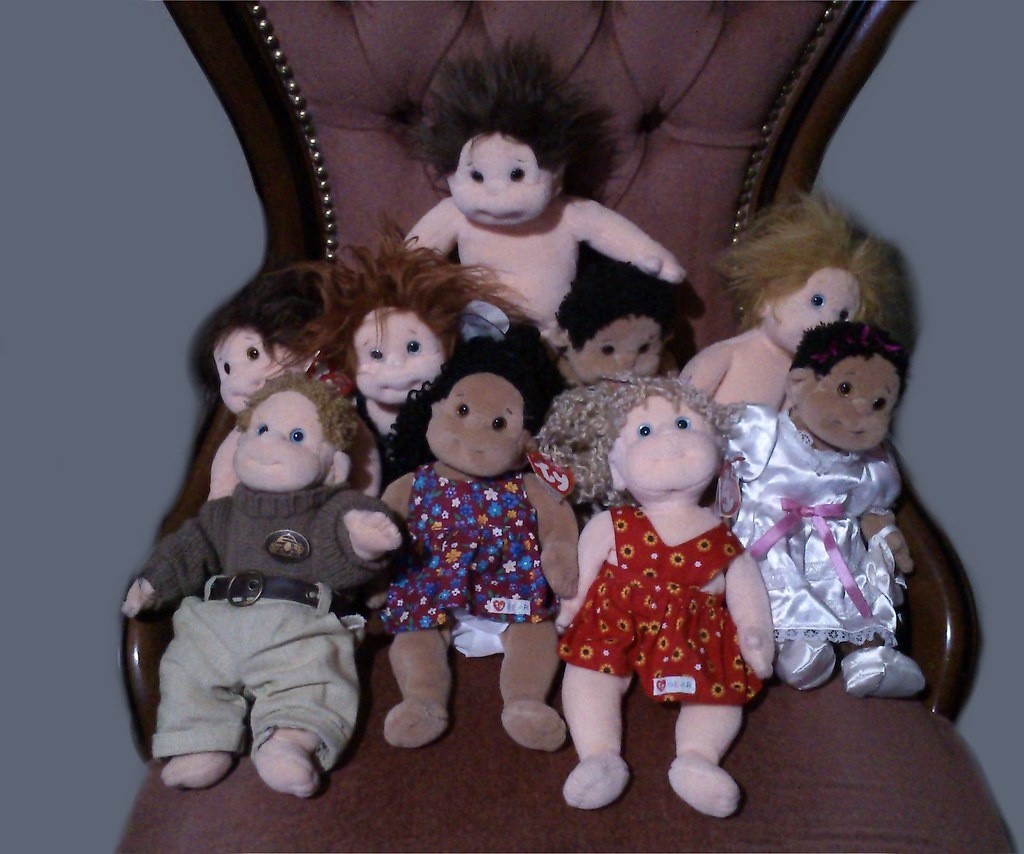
[678,187,905,408]
[368,334,581,755]
[716,317,928,701]
[554,236,685,382]
[530,372,780,821]
[119,365,405,801]
[392,37,686,349]
[183,254,381,520]
[305,229,538,439]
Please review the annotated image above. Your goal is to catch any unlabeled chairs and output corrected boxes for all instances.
[116,0,1017,853]
